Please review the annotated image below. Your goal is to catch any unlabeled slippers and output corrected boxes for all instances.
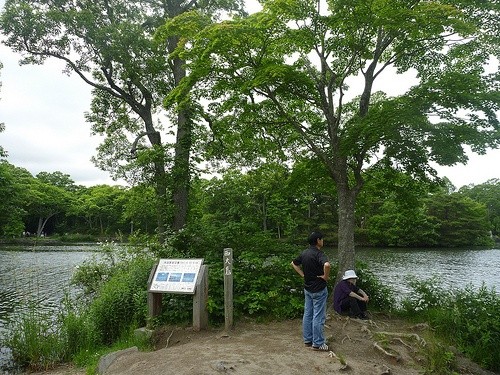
[304,341,313,347]
[311,344,333,352]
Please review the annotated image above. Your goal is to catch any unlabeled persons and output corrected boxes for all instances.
[291,230,331,352]
[334,270,369,320]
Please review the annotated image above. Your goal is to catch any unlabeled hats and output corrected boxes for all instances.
[342,270,358,280]
